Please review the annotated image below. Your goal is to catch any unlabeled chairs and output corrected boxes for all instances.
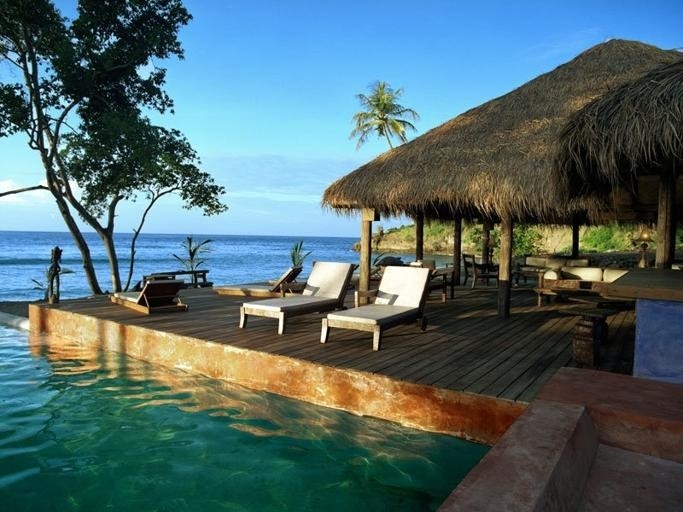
[216,265,303,297]
[110,280,189,315]
[239,260,356,336]
[461,253,499,289]
[319,265,434,352]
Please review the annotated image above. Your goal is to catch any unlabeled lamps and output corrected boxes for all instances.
[631,224,654,269]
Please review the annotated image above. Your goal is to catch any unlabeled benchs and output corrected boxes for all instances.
[559,304,632,365]
[150,269,214,289]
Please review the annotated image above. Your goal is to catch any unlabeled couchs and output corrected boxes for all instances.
[512,253,635,309]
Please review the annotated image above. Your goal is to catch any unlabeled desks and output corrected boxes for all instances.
[603,267,683,385]
[351,258,457,302]
